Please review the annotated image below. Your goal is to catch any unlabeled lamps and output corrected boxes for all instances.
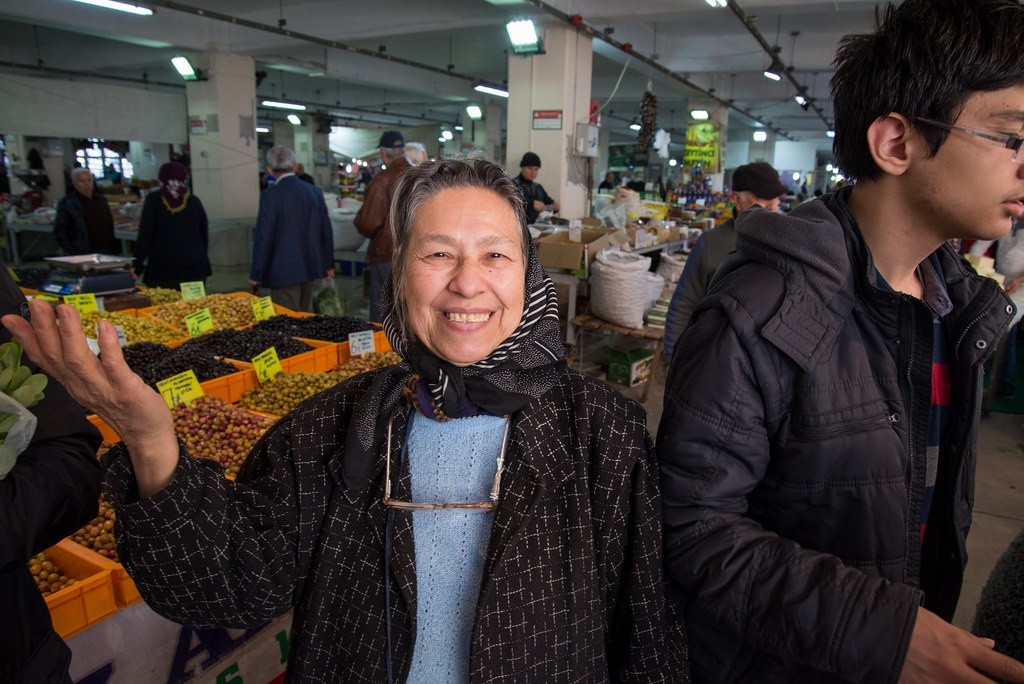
[171,54,203,80]
[440,14,546,142]
[630,56,816,142]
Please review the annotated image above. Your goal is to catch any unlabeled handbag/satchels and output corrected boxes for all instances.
[313,275,345,317]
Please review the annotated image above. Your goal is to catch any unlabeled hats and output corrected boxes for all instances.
[732,161,795,199]
[518,152,541,168]
[158,161,190,182]
[375,130,403,148]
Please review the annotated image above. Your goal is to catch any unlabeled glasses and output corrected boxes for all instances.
[382,406,511,513]
[906,116,1024,166]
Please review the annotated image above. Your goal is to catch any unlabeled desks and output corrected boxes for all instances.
[7,203,139,264]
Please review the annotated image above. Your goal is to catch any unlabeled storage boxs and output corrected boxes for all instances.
[41,292,392,638]
[536,206,694,387]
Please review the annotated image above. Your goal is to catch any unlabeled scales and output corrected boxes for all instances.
[39,254,138,296]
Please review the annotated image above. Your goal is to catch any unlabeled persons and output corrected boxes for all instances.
[0,259,104,684]
[512,153,560,223]
[0,161,692,684]
[657,175,676,200]
[130,162,212,287]
[655,0,1023,683]
[623,169,646,191]
[662,163,794,377]
[356,131,415,322]
[248,146,335,312]
[792,182,830,209]
[598,172,614,194]
[55,167,120,255]
[967,215,1024,401]
